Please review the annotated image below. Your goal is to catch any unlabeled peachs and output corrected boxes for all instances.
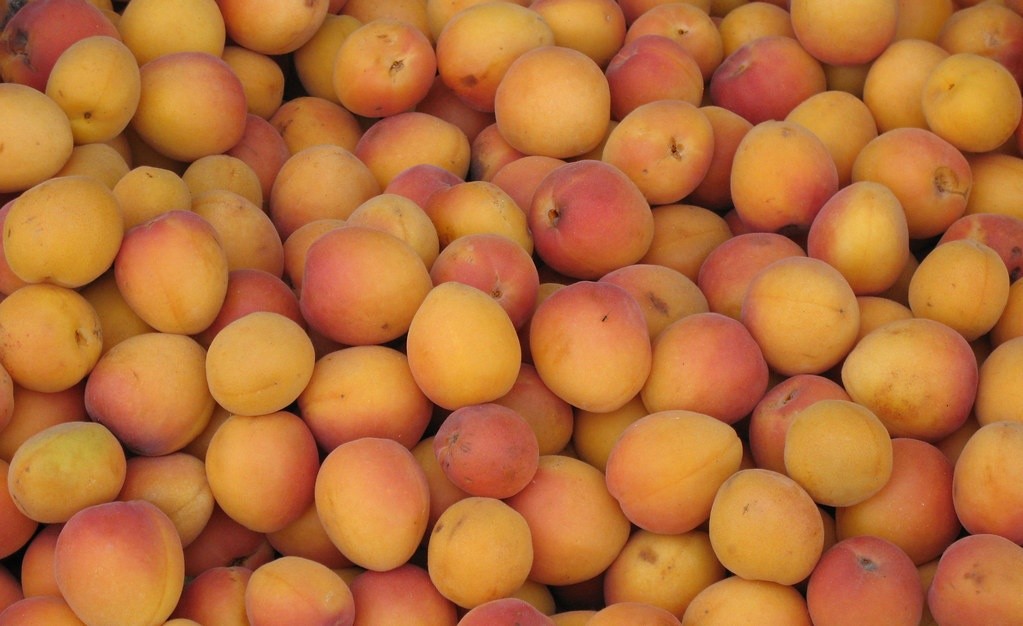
[0,0,1023,626]
